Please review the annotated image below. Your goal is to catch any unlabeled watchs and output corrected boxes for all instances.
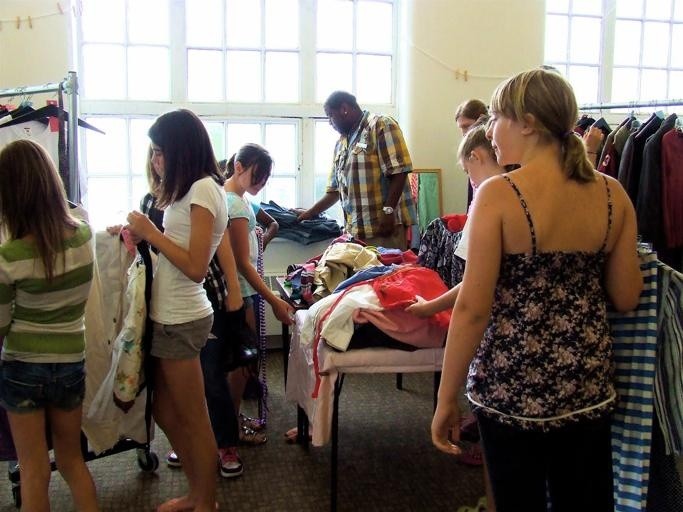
[381,206,395,216]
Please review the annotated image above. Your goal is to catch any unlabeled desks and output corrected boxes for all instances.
[274,275,445,507]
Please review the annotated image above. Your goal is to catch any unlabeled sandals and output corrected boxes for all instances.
[238,428,267,445]
[238,414,266,428]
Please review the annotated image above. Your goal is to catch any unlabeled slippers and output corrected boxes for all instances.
[463,441,482,465]
[459,412,477,433]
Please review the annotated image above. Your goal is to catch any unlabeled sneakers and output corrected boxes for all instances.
[219,447,243,477]
[166,449,181,467]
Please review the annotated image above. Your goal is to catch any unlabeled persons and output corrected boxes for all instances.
[0,138,100,512]
[402,68,645,512]
[105,111,296,510]
[288,90,418,250]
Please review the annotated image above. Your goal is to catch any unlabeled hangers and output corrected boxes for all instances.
[1,79,104,138]
[580,103,682,135]
